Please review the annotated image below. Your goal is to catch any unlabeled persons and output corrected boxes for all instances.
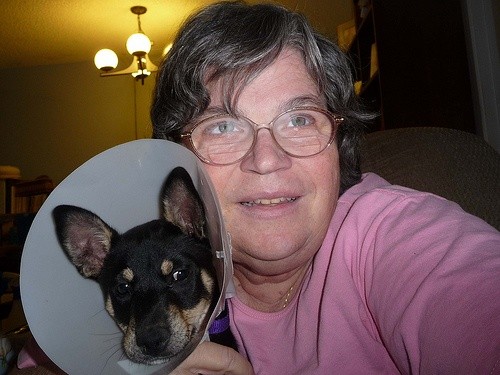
[8,0,500,375]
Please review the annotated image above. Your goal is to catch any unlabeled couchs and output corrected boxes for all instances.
[356,126,500,235]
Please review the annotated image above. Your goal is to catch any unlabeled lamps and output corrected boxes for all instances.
[92,6,174,85]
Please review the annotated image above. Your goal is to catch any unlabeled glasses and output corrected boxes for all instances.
[180,106,344,166]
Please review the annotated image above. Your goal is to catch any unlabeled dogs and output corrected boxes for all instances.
[51,167,216,366]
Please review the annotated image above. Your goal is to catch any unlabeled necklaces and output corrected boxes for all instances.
[283,287,294,307]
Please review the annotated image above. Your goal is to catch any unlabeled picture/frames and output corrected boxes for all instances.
[336,19,380,96]
